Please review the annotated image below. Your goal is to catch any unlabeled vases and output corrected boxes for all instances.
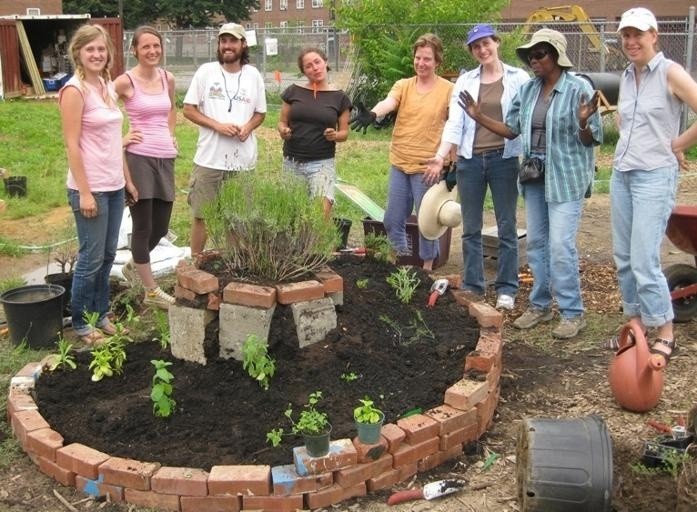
[44,273,74,318]
[332,217,352,252]
[3,176,27,198]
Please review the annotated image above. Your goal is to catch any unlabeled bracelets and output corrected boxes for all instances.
[436,152,445,160]
[577,122,588,132]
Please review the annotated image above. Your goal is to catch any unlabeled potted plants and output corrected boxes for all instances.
[354,399,385,444]
[267,391,333,457]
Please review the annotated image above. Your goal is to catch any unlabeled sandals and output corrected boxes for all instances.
[603,329,648,349]
[650,338,679,363]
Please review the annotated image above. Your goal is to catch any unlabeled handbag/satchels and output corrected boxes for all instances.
[519,157,545,183]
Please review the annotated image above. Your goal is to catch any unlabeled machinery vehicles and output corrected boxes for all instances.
[515,6,607,69]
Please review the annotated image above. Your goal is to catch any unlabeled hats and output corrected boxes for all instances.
[516,28,573,67]
[418,180,463,240]
[467,24,494,44]
[617,8,658,32]
[218,23,247,39]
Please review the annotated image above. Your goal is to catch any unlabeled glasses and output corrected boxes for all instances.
[526,52,545,61]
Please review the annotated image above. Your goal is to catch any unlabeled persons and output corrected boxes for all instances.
[279,48,353,222]
[348,33,456,271]
[111,26,178,309]
[457,28,603,339]
[419,24,531,310]
[57,23,139,347]
[183,22,267,258]
[608,7,697,365]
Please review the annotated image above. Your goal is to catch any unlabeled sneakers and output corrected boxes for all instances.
[81,322,129,345]
[495,294,513,309]
[552,316,586,339]
[122,261,142,286]
[513,307,554,329]
[143,286,176,310]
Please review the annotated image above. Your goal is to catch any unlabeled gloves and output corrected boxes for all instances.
[443,161,457,191]
[348,102,376,134]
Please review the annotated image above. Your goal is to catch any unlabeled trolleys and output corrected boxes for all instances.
[662,205,697,322]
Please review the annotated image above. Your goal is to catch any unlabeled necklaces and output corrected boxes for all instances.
[220,68,242,113]
